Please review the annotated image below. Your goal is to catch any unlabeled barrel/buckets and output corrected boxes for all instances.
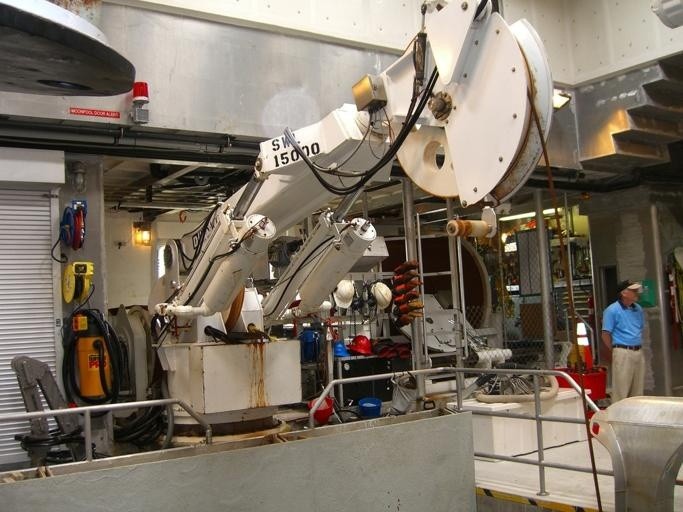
[300,329,318,361]
[306,398,336,426]
[359,397,381,420]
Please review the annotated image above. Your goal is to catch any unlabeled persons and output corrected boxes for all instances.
[602,279,645,403]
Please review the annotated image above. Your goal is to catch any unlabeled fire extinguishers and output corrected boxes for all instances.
[586,290,595,323]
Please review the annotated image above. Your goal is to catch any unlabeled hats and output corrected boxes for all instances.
[616,279,642,294]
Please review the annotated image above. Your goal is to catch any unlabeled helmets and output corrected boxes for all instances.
[332,278,355,309]
[333,340,352,357]
[371,282,392,310]
[346,334,374,355]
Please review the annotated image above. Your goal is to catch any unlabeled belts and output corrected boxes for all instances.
[612,344,642,351]
